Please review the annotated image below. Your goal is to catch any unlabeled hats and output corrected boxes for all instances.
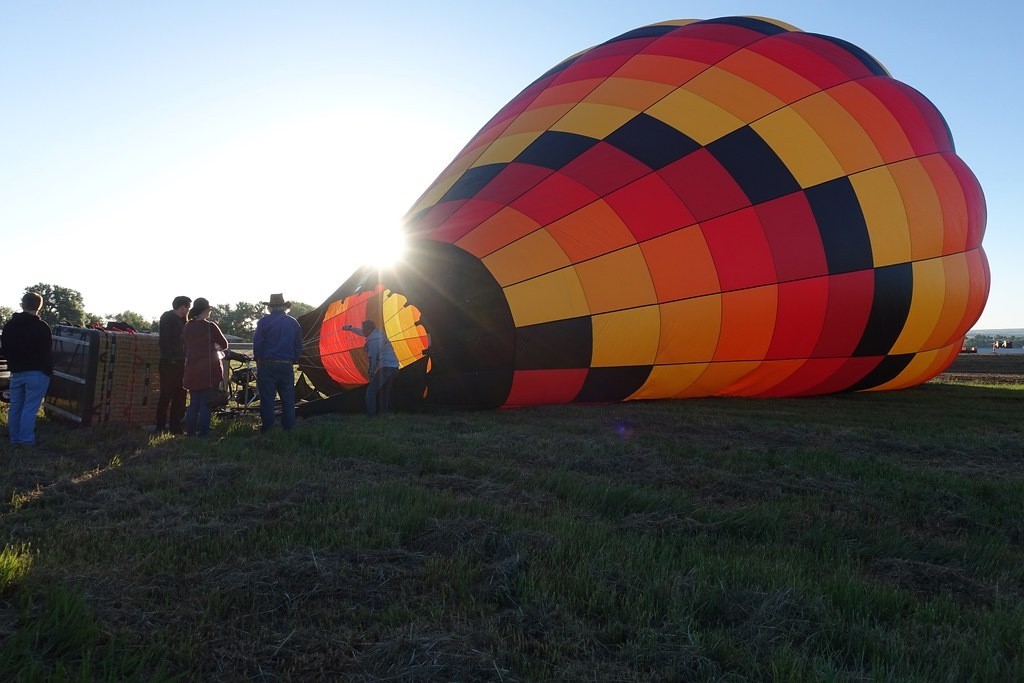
[258,293,292,309]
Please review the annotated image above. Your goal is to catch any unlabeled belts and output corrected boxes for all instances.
[265,358,290,363]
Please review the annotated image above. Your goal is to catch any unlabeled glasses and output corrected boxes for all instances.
[207,305,214,310]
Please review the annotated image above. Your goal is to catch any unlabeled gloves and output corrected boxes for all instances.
[342,324,352,331]
[368,376,373,384]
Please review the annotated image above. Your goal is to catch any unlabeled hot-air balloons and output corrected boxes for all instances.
[39,14,992,432]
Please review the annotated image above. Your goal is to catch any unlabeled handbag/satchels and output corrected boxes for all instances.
[207,388,230,408]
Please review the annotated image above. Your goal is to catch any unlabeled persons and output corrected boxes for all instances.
[1,293,55,444]
[180,298,230,438]
[968,349,970,354]
[154,296,191,434]
[253,293,303,434]
[342,320,400,417]
